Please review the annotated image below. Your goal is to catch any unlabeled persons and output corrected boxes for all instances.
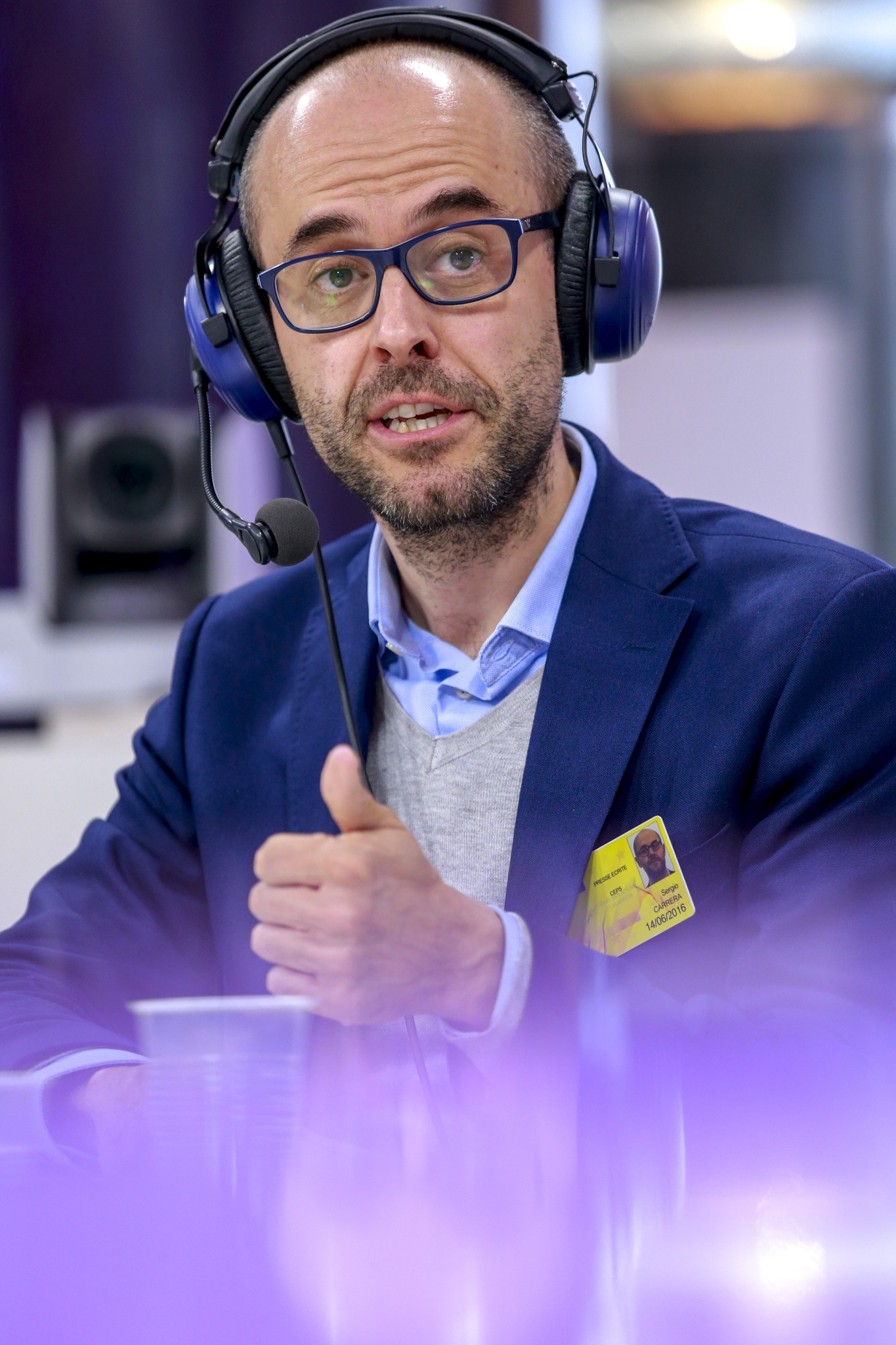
[0,35,895,1157]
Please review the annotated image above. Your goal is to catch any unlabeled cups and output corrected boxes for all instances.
[127,990,316,1213]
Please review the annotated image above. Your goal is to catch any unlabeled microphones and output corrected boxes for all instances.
[187,352,320,566]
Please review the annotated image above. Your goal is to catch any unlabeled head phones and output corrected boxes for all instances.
[182,7,667,423]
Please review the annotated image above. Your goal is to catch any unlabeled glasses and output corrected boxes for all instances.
[636,840,662,858]
[256,207,566,334]
